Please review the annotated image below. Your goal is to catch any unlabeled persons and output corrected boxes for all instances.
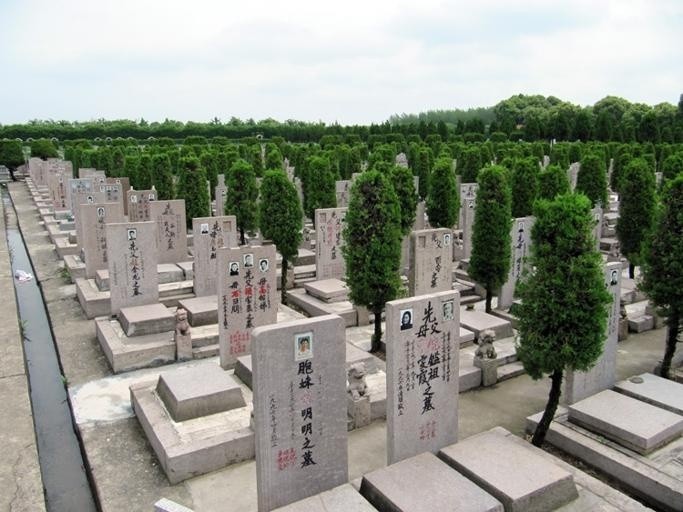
[297,337,312,358]
[260,260,269,272]
[88,196,93,202]
[98,208,103,215]
[244,255,253,267]
[518,227,526,242]
[202,224,208,234]
[230,263,238,275]
[444,234,451,245]
[149,194,154,200]
[595,215,599,222]
[610,270,619,286]
[128,230,136,241]
[401,311,413,330]
[443,301,453,322]
